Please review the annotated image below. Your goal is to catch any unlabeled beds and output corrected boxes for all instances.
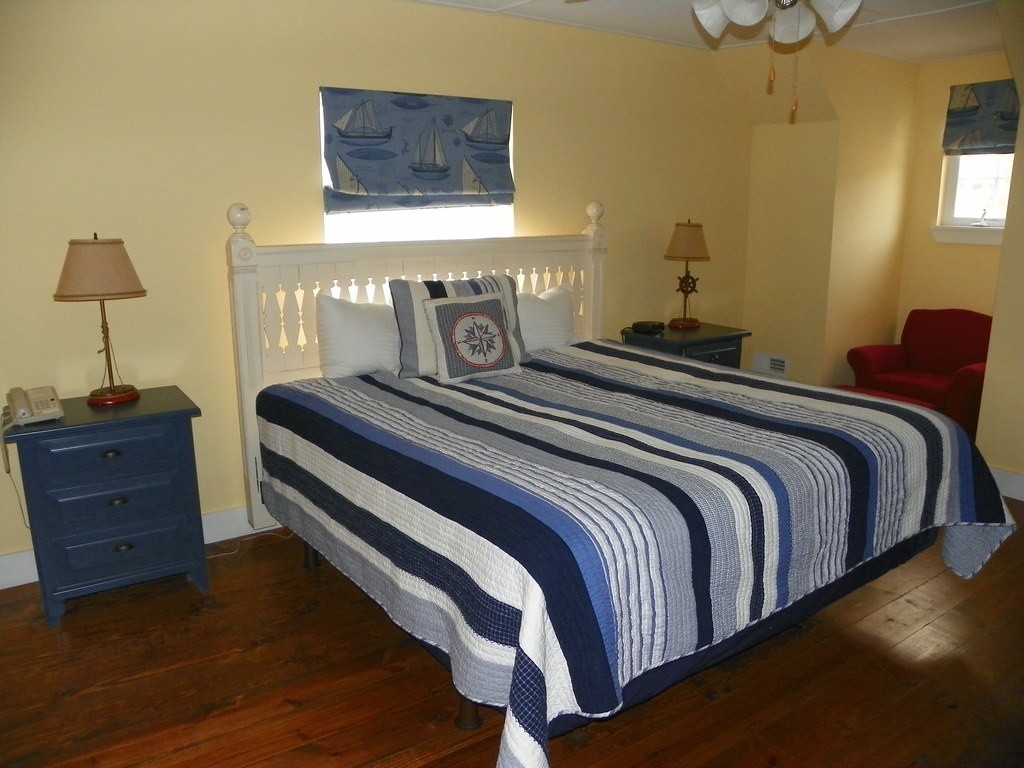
[225,203,1016,768]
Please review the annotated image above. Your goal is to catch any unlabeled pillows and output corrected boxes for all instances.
[315,293,401,377]
[522,285,573,353]
[424,293,522,383]
[388,276,531,385]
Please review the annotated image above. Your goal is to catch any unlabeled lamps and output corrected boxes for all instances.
[52,233,146,410]
[665,219,711,328]
[691,0,860,114]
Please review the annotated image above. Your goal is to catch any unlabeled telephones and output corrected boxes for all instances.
[7,383,64,428]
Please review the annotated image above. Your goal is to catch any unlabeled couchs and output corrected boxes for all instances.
[846,308,993,434]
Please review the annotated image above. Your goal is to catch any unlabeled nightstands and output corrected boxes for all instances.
[0,384,211,629]
[621,322,752,370]
